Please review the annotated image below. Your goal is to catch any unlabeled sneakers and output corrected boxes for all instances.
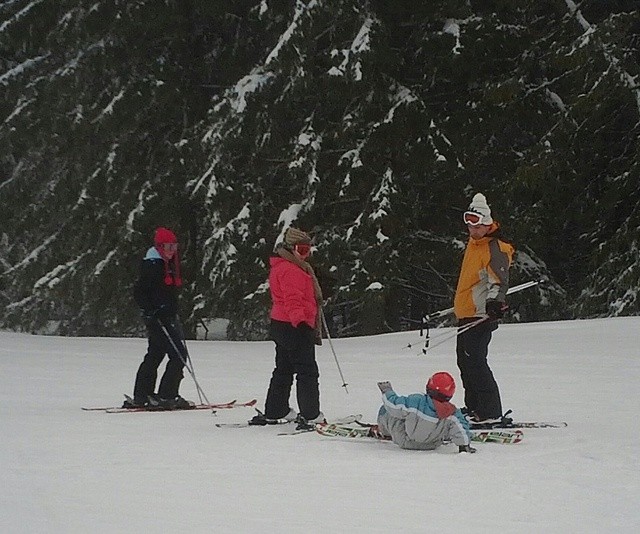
[164,396,195,408]
[265,409,298,423]
[461,407,472,414]
[465,414,502,424]
[371,426,392,440]
[134,395,167,406]
[296,412,327,428]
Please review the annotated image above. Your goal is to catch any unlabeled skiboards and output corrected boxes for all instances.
[463,418,568,430]
[315,418,524,445]
[80,398,257,414]
[216,410,362,435]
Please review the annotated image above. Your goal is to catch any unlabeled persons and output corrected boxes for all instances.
[133,227,189,410]
[455,193,514,426]
[370,372,477,453]
[265,228,328,425]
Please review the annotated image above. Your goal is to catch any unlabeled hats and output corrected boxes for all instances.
[154,227,181,286]
[286,228,312,251]
[427,372,455,396]
[469,193,493,225]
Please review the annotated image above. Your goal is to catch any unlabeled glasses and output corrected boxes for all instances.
[464,212,483,225]
[162,243,177,251]
[296,245,310,256]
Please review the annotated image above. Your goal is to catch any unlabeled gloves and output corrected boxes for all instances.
[378,382,392,392]
[459,445,477,453]
[486,301,505,319]
[300,323,317,344]
